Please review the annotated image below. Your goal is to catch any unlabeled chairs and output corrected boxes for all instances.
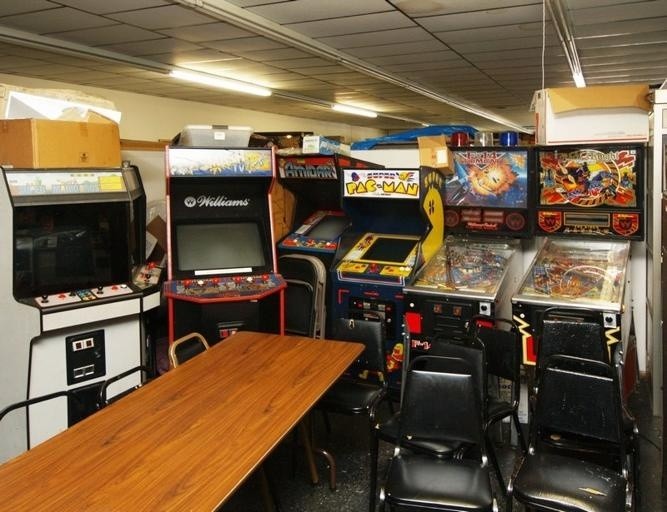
[504,307,639,511]
[370,313,527,511]
[310,307,395,459]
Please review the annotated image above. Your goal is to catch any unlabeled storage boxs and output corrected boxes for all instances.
[417,132,454,175]
[1,109,122,171]
[179,123,253,149]
[527,82,652,146]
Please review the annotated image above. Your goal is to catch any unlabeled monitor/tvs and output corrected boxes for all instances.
[342,232,423,267]
[173,215,270,280]
[13,200,129,299]
[302,215,352,242]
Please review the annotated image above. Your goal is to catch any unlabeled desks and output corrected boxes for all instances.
[2,329,365,511]
